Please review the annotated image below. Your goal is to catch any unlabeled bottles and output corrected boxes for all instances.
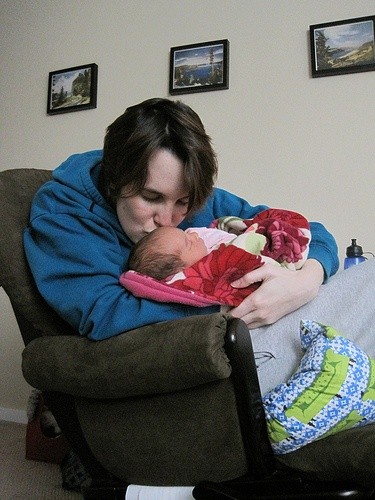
[344,238,375,271]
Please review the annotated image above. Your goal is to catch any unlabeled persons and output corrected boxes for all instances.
[25,97,375,500]
[125,209,312,308]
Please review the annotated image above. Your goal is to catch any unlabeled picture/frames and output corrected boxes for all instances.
[47,63,98,115]
[169,39,230,95]
[309,15,375,78]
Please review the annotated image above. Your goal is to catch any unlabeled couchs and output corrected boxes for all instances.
[0,168,375,500]
